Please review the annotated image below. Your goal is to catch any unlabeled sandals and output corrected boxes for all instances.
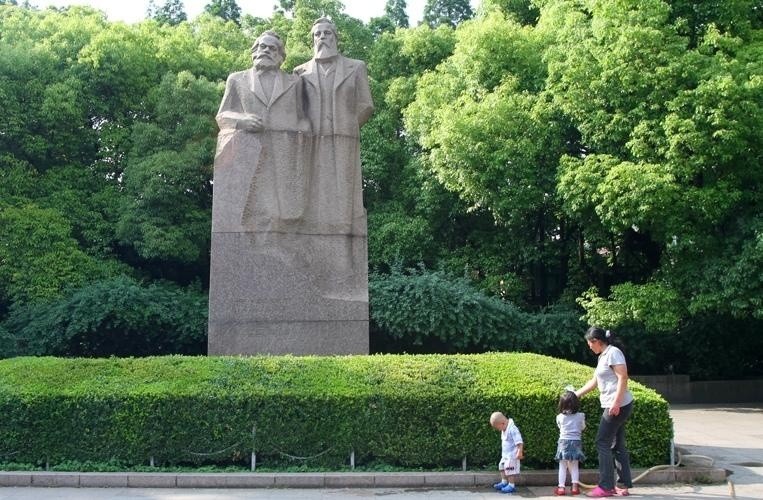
[501,485,518,492]
[586,485,629,497]
[494,482,508,490]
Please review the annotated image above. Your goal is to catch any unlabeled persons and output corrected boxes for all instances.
[489,411,524,493]
[555,325,634,497]
[215,17,375,136]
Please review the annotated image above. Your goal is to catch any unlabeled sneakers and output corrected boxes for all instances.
[554,486,566,495]
[571,488,580,495]
